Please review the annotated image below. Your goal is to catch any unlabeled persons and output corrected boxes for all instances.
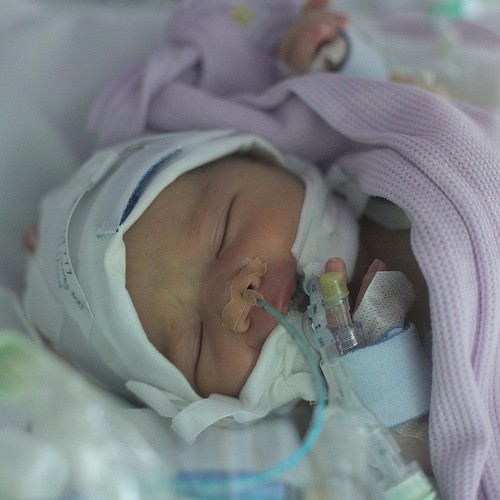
[19,1,499,499]
